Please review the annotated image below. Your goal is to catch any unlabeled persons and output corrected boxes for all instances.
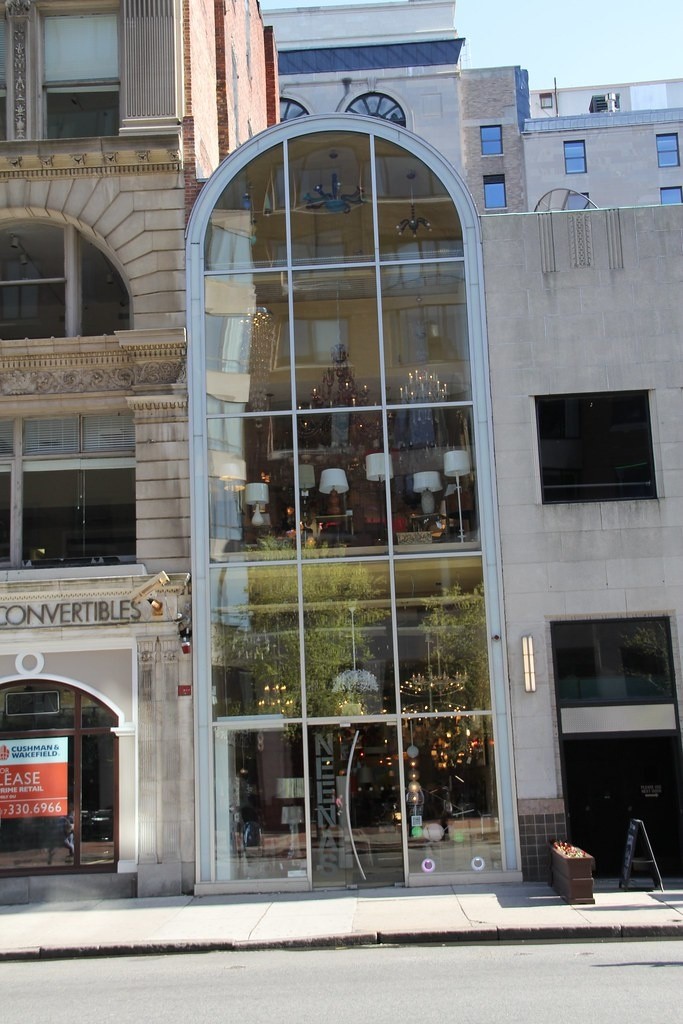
[59,806,74,856]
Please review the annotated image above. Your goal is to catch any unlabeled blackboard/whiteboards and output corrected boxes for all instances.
[619,819,663,886]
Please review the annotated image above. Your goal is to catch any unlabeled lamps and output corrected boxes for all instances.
[218,609,489,844]
[11,238,20,247]
[146,598,162,612]
[522,636,537,692]
[107,273,114,284]
[240,151,471,547]
[20,254,28,265]
[121,294,128,306]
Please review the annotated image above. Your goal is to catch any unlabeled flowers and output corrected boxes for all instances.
[554,840,586,857]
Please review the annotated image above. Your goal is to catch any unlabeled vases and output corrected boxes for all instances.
[550,844,595,904]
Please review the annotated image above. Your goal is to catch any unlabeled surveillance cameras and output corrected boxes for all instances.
[493,634,500,641]
